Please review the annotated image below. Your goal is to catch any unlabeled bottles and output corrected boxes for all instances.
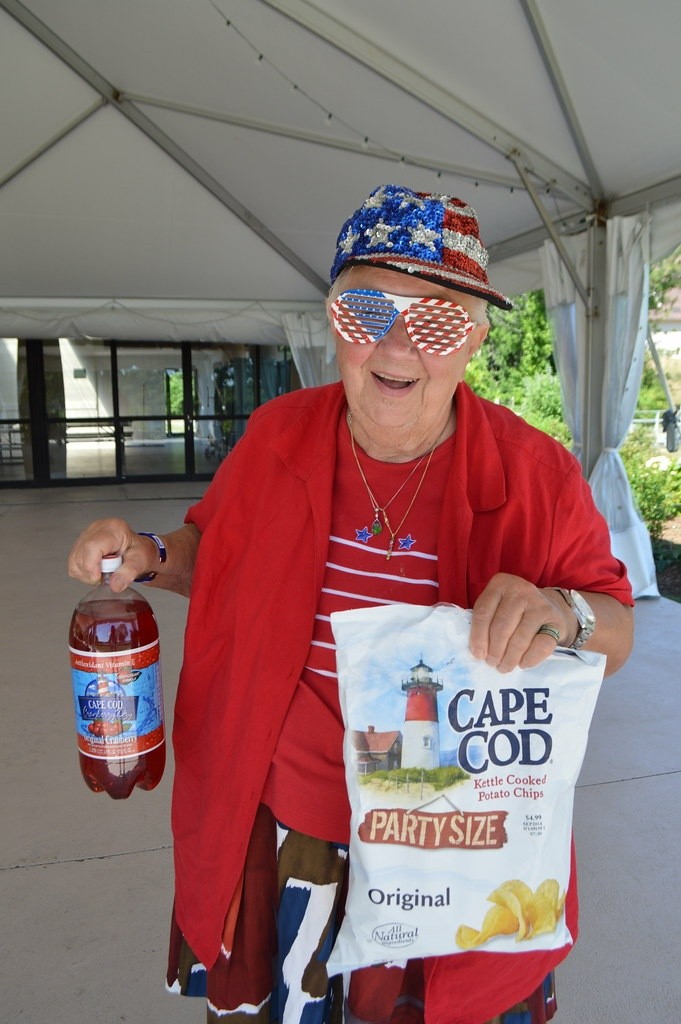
[69,553,166,800]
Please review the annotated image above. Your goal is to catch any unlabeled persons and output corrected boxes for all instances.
[67,182,635,1024]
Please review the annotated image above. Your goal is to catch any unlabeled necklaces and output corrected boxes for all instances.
[344,403,453,560]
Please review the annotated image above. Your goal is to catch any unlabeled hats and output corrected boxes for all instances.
[332,186,513,312]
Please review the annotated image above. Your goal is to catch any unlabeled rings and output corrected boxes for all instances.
[537,624,561,644]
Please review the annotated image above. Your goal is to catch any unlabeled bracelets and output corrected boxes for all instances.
[132,530,167,583]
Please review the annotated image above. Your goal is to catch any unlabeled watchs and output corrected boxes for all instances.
[544,586,596,650]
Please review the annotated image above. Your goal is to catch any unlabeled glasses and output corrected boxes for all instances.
[331,289,480,356]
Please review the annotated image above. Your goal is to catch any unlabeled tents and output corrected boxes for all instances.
[1,0,679,599]
